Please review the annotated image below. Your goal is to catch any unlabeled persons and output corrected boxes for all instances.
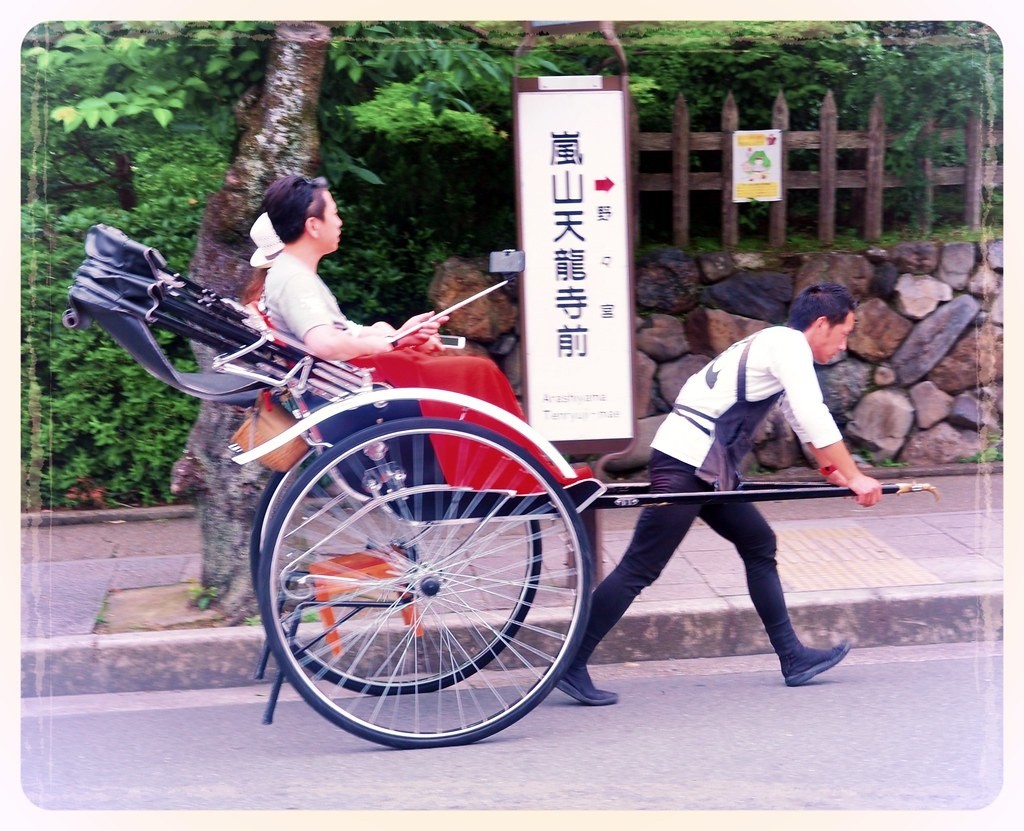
[263,174,450,368]
[246,213,445,354]
[554,283,882,706]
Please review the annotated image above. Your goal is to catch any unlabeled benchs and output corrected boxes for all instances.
[253,302,594,516]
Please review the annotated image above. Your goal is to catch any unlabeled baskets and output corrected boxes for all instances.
[226,405,310,471]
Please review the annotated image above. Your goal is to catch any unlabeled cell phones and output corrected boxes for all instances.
[489,251,525,273]
[439,335,466,349]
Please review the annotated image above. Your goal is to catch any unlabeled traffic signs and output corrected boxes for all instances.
[512,93,641,456]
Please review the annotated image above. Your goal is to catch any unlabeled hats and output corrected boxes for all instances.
[249,212,287,268]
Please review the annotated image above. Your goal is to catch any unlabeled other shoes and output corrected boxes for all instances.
[780,640,850,686]
[544,655,618,705]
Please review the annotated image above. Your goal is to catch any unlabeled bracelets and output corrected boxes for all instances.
[820,464,838,475]
[386,336,398,347]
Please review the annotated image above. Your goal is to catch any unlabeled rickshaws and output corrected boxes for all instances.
[60,222,941,749]
